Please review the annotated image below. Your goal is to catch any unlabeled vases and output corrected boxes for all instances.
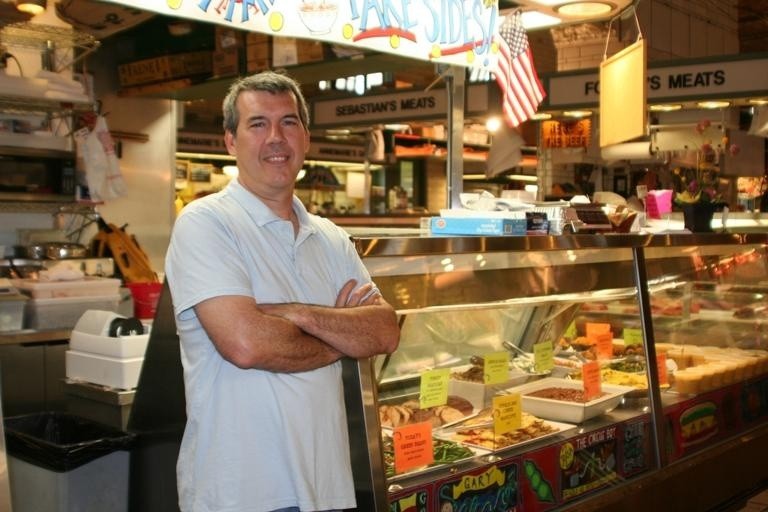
[680,202,714,230]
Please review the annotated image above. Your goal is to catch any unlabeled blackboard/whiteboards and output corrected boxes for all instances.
[599,39,648,151]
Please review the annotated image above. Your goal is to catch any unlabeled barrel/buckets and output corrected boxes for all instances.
[128,282,166,319]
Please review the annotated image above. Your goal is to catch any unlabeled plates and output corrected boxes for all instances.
[445,363,635,424]
[381,411,578,482]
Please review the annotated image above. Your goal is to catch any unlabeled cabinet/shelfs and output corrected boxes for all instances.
[345,220,767,511]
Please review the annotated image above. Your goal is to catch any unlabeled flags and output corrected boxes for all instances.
[493,8,547,130]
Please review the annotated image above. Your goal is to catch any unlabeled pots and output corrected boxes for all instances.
[15,244,56,260]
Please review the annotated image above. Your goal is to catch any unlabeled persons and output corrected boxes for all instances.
[165,70,400,512]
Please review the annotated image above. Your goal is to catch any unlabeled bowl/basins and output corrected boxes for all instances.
[0,265,48,280]
[39,242,87,260]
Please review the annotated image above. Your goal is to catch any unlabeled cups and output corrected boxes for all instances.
[674,349,768,398]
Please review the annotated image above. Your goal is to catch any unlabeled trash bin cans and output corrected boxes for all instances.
[3,411,135,512]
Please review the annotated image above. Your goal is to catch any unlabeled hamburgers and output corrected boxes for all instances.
[680,402,719,447]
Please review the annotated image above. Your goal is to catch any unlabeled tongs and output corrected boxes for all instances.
[556,351,588,366]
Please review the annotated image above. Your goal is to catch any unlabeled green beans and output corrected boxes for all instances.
[524,460,555,502]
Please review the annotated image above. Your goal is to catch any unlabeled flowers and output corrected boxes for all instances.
[672,117,740,201]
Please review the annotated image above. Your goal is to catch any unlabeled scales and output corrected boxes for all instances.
[523,202,613,234]
